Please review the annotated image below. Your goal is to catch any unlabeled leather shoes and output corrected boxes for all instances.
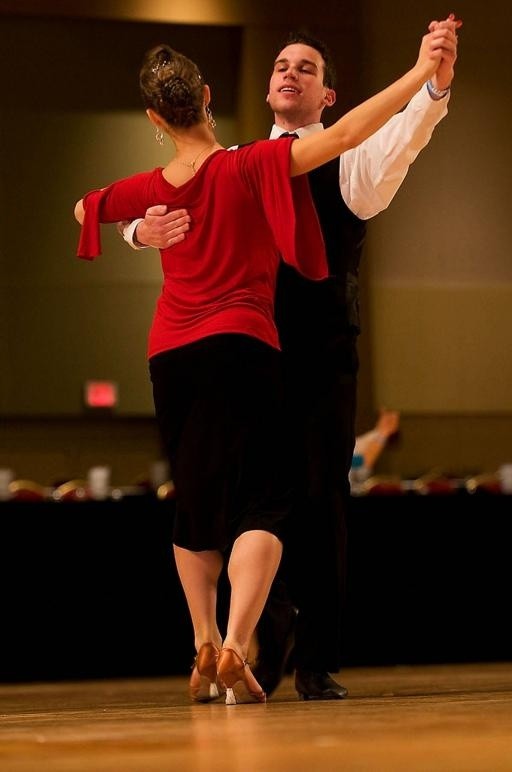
[250,607,296,696]
[292,672,348,700]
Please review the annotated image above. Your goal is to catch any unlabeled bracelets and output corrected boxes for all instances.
[426,79,449,97]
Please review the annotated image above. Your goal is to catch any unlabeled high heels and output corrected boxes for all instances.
[216,646,266,706]
[188,642,220,705]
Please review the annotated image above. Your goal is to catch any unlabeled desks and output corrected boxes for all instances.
[1,489,512,684]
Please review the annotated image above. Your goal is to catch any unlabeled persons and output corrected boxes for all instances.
[115,21,462,699]
[347,403,402,487]
[68,11,465,710]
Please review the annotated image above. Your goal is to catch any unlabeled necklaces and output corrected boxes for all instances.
[167,140,218,183]
